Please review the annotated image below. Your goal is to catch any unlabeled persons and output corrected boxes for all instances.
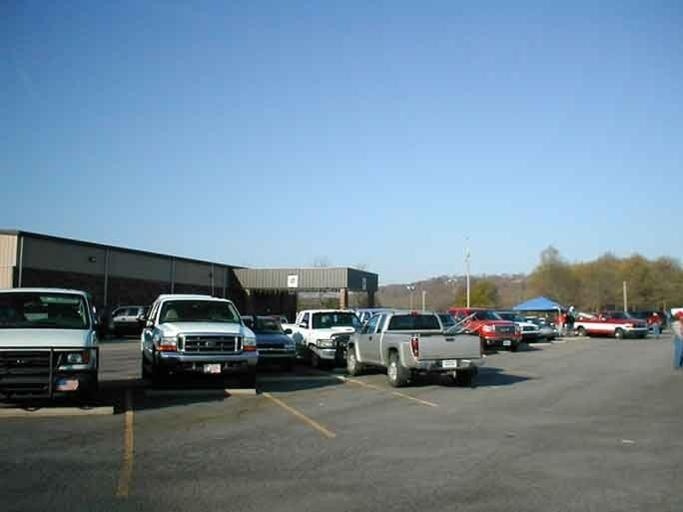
[564,306,576,337]
[669,311,682,371]
[647,310,661,339]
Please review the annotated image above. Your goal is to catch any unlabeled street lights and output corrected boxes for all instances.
[464,246,470,307]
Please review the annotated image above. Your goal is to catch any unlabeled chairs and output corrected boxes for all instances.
[164,308,179,321]
[320,315,332,328]
[0,307,24,321]
[210,309,225,320]
